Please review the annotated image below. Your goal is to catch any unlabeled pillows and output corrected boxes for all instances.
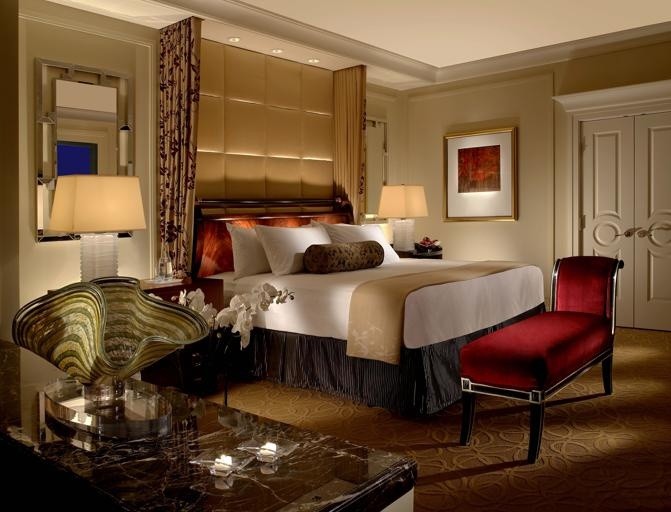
[225,219,401,275]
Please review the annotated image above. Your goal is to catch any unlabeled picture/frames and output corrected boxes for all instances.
[441,125,519,222]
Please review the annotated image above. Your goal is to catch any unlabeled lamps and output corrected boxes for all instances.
[49,172,148,283]
[377,182,430,252]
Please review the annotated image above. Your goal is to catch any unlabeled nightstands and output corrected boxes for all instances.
[130,273,226,398]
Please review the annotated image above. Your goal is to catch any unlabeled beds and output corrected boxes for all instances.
[191,187,545,422]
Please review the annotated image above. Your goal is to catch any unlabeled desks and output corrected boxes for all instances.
[0,387,414,512]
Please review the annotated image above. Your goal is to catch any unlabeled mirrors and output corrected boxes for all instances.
[364,113,389,216]
[33,57,138,244]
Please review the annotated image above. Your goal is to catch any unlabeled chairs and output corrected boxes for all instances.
[457,253,624,462]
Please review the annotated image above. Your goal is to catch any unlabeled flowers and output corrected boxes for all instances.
[149,280,296,350]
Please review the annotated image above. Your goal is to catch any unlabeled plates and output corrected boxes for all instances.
[236,436,299,464]
[189,446,256,477]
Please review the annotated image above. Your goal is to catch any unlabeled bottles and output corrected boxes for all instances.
[157,240,173,283]
